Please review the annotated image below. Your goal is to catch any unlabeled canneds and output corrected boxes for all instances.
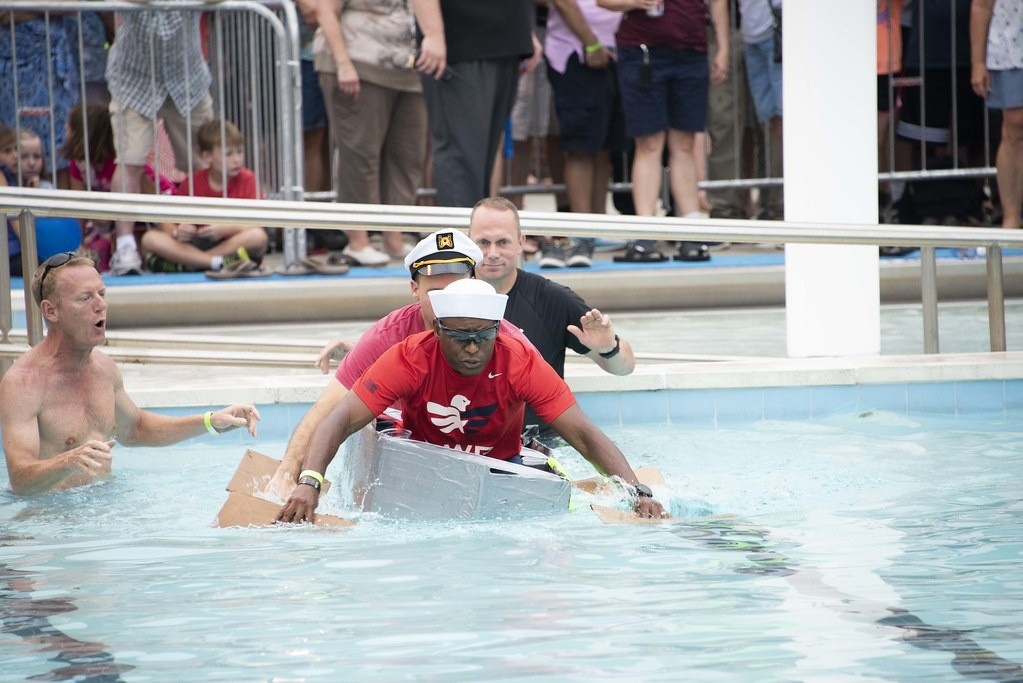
[646,0,664,17]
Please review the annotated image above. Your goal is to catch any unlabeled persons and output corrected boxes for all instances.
[447,0,1023,270]
[265,225,673,525]
[0,250,260,498]
[0,0,447,281]
[315,197,636,455]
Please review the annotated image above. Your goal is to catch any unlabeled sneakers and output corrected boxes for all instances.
[110,248,141,275]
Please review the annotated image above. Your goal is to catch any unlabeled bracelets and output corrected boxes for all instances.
[298,469,323,493]
[171,223,180,239]
[586,42,603,53]
[203,411,220,436]
[599,334,620,359]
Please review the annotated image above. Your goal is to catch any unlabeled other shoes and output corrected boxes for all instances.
[387,244,412,259]
[343,244,394,265]
[570,234,630,251]
[145,252,183,271]
[676,239,729,252]
[219,255,241,273]
[567,240,592,268]
[521,234,549,257]
[541,240,565,267]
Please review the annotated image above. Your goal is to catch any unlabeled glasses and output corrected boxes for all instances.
[434,317,500,342]
[39,249,81,300]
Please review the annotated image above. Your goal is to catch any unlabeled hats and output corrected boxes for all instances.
[405,226,484,279]
[427,279,509,323]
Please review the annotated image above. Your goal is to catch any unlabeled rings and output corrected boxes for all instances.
[660,511,667,515]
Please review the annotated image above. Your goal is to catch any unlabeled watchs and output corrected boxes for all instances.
[633,483,653,497]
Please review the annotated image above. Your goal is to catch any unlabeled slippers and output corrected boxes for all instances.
[613,243,668,262]
[674,240,711,261]
[206,258,272,279]
[277,255,350,274]
